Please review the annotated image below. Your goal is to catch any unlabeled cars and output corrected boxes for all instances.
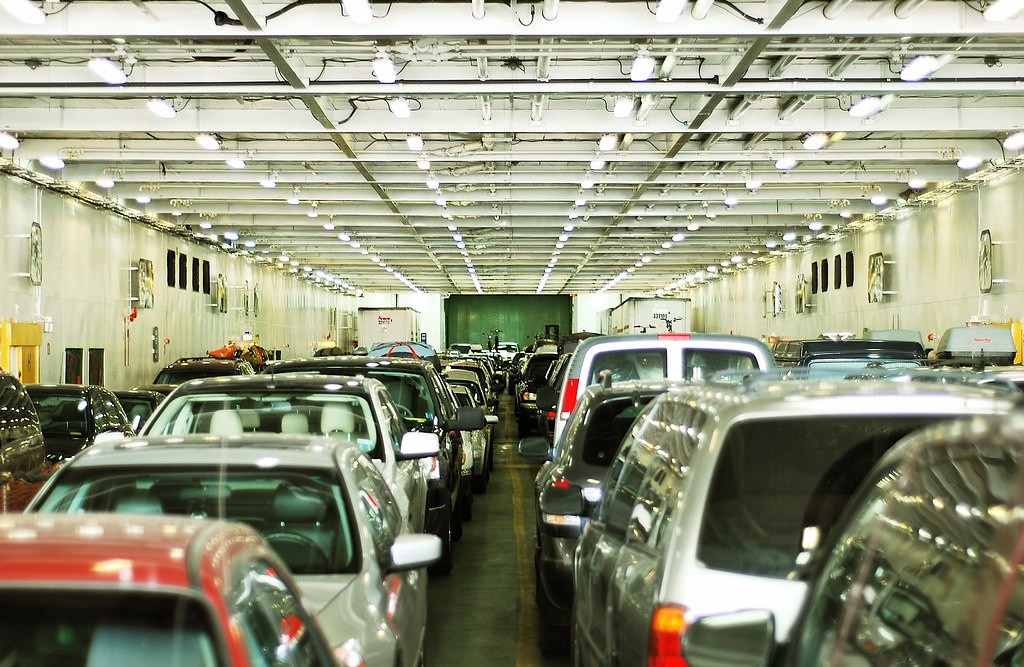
[518,367,686,656]
[20,382,135,468]
[0,510,339,667]
[19,429,445,667]
[106,327,1024,574]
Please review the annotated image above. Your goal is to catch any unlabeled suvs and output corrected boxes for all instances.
[680,404,1023,667]
[90,375,441,533]
[0,367,48,514]
[573,365,1023,666]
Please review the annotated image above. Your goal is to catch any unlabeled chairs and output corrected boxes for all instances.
[209,409,242,435]
[113,490,163,515]
[281,412,309,436]
[85,614,218,666]
[320,401,354,437]
[236,409,261,432]
[261,480,336,565]
